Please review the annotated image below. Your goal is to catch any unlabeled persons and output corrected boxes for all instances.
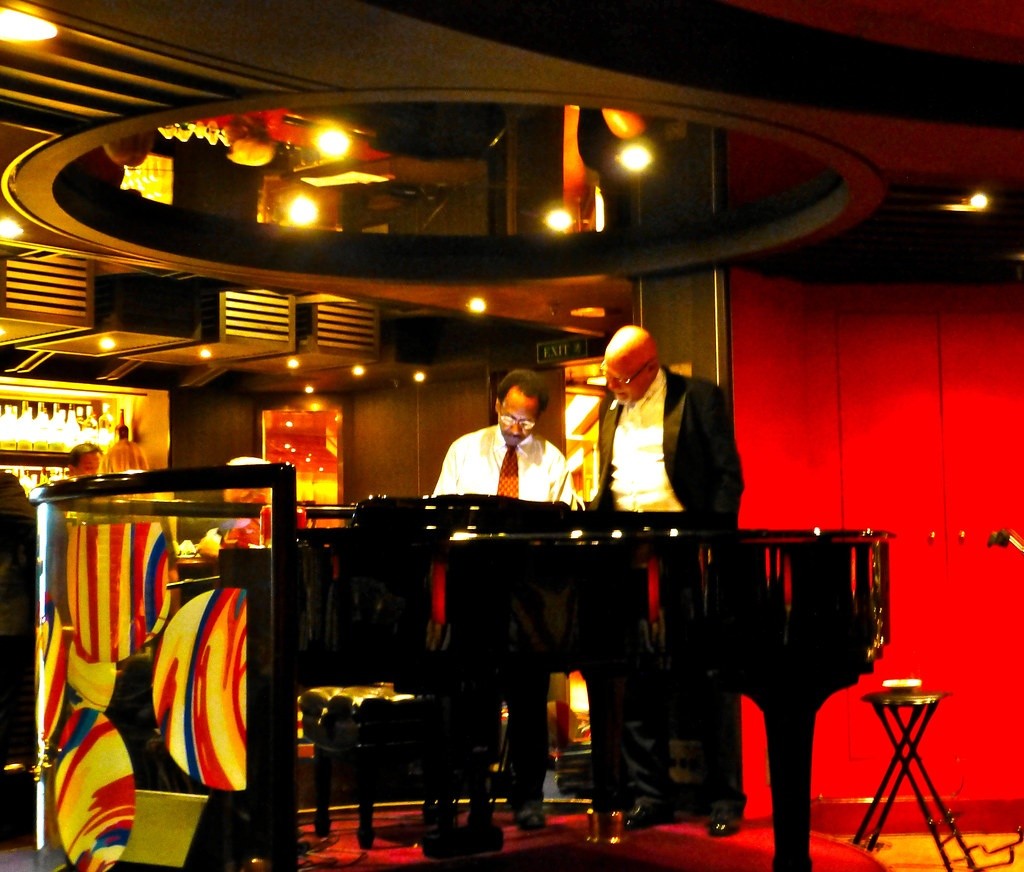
[67,442,104,477]
[432,369,574,830]
[589,324,747,835]
[198,457,271,557]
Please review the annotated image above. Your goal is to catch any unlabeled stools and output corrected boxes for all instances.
[853,691,976,871]
[300,684,451,851]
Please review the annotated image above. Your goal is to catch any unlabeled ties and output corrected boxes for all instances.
[497,444,519,499]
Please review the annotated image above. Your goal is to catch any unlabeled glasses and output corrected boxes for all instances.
[599,360,646,385]
[499,404,536,431]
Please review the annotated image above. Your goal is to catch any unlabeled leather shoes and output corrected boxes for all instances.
[709,802,730,834]
[513,806,545,829]
[623,804,674,830]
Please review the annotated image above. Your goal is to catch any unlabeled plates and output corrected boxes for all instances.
[882,678,922,689]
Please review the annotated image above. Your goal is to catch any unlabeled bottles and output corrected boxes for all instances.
[0,401,140,498]
